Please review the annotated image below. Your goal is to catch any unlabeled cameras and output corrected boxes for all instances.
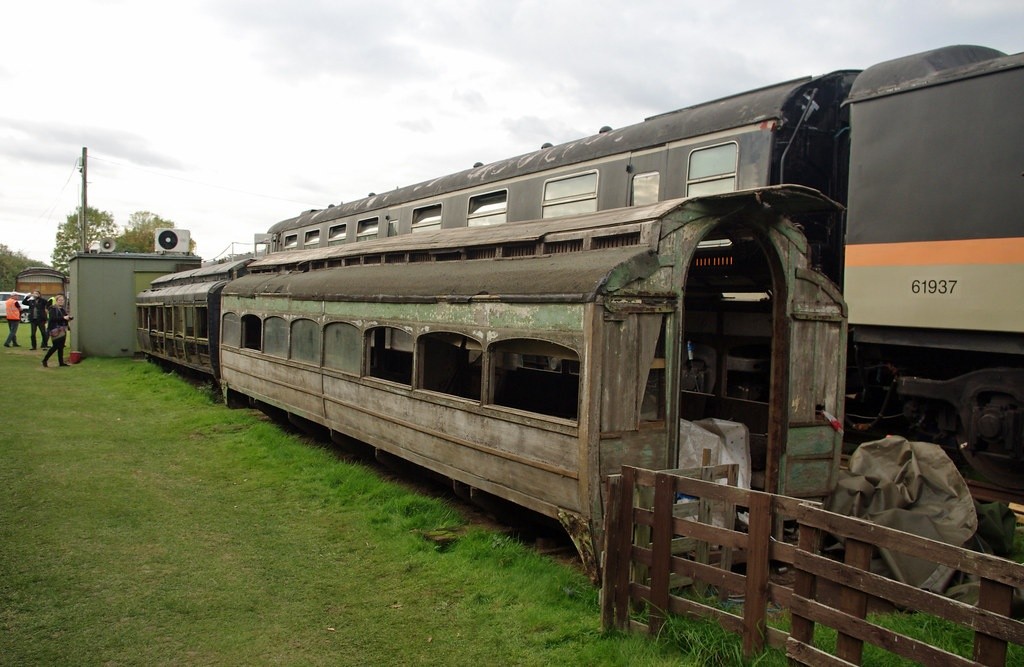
[69,317,73,320]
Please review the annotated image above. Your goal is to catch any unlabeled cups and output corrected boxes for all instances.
[69,316,73,320]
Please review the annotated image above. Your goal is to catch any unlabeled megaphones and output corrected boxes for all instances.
[100,238,116,253]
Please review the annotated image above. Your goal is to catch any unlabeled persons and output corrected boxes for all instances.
[41,295,73,367]
[44,292,67,348]
[4,292,22,348]
[22,290,48,350]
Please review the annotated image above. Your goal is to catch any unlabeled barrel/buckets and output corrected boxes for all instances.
[69,351,81,364]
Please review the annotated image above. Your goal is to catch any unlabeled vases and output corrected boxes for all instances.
[70,350,82,363]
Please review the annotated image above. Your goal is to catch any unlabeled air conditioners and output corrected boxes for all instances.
[154,228,190,256]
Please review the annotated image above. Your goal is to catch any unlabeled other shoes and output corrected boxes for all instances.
[28,347,36,350]
[4,343,11,347]
[41,360,48,368]
[58,363,71,367]
[13,343,21,347]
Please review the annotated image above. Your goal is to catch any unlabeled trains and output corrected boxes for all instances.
[264,42,1024,493]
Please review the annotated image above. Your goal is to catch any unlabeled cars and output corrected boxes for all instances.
[0,291,31,324]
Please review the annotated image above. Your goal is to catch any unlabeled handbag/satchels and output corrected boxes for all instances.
[49,326,67,340]
